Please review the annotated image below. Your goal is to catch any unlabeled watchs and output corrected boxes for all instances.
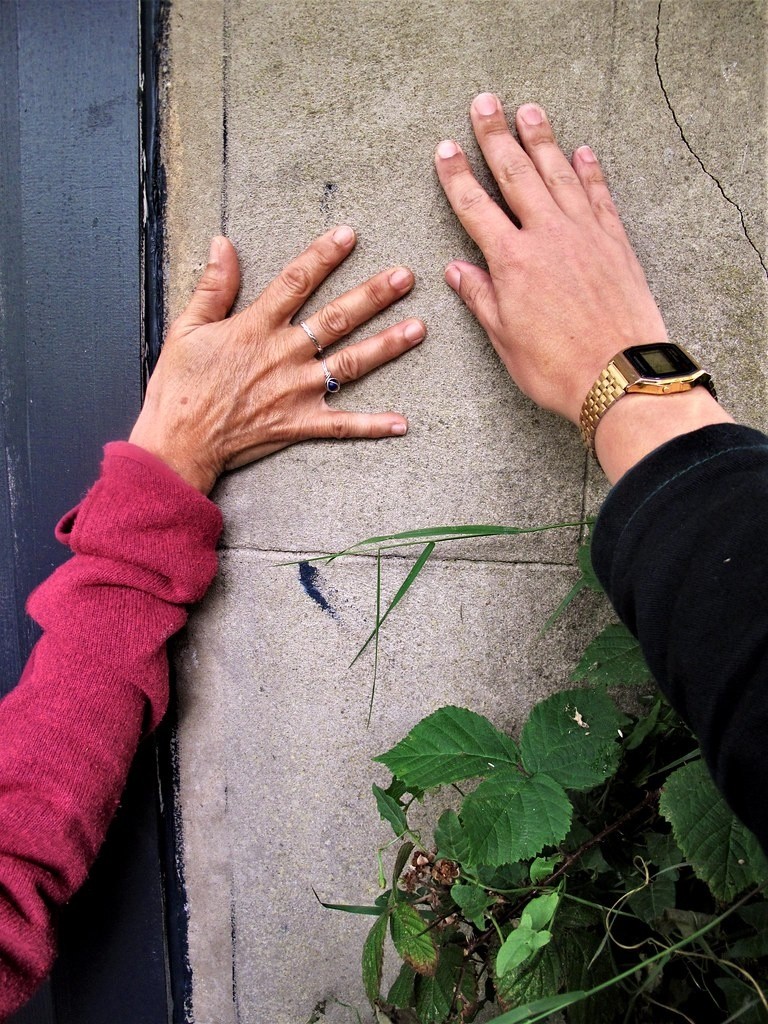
[581,339,721,466]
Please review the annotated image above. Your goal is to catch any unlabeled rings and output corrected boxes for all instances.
[321,357,340,394]
[299,320,322,354]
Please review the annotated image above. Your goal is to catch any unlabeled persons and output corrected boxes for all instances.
[0,217,427,1024]
[434,93,768,860]
